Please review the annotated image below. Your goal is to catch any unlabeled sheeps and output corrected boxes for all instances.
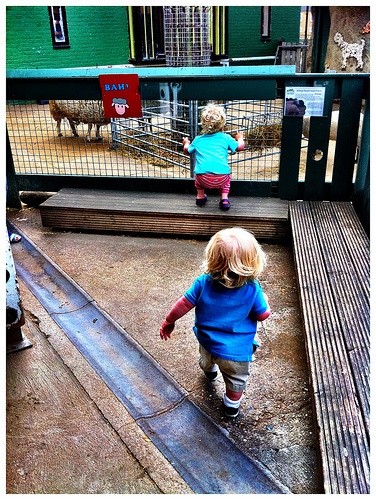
[49,98,118,143]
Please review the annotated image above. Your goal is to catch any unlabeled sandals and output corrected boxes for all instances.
[196,194,207,205]
[219,198,230,210]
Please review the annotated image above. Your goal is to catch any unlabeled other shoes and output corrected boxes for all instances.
[205,371,218,380]
[225,407,239,418]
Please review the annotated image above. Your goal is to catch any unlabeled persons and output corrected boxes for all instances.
[183,104,244,208]
[160,228,271,418]
